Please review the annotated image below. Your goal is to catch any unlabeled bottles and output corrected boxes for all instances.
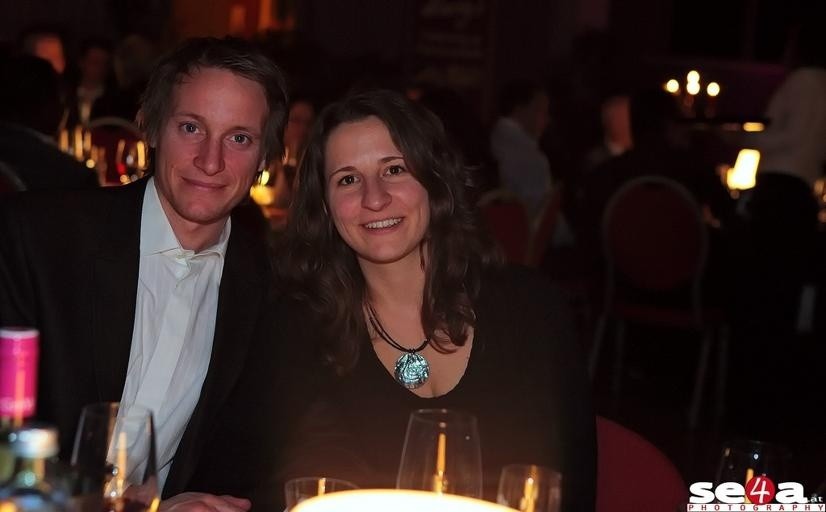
[0,428,72,511]
[1,324,43,482]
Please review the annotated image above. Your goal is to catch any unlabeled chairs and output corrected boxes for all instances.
[477,177,728,511]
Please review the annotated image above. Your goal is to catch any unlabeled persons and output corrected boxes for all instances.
[284,89,598,512]
[0,53,826,316]
[0,36,310,512]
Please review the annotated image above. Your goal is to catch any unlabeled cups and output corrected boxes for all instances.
[73,402,161,512]
[284,476,363,512]
[395,407,485,502]
[496,462,565,512]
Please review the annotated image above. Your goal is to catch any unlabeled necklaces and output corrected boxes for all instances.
[366,302,444,390]
